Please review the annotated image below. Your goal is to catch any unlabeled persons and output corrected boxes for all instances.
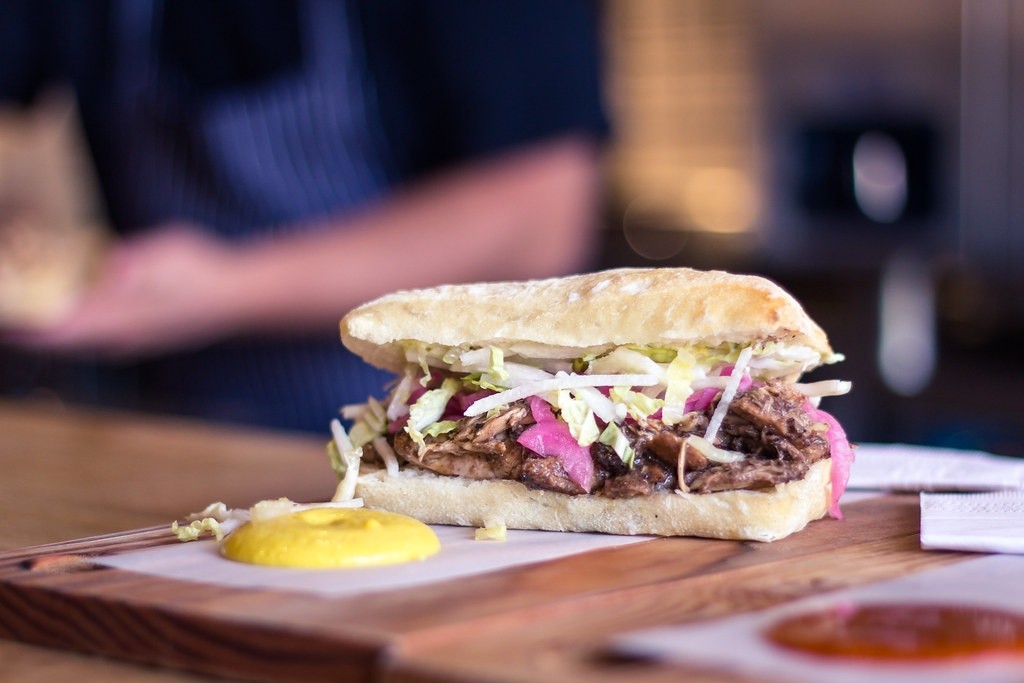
[1,0,620,443]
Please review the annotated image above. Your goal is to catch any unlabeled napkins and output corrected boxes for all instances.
[918,492,1024,554]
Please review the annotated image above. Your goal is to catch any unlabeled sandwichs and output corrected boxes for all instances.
[324,268,854,543]
